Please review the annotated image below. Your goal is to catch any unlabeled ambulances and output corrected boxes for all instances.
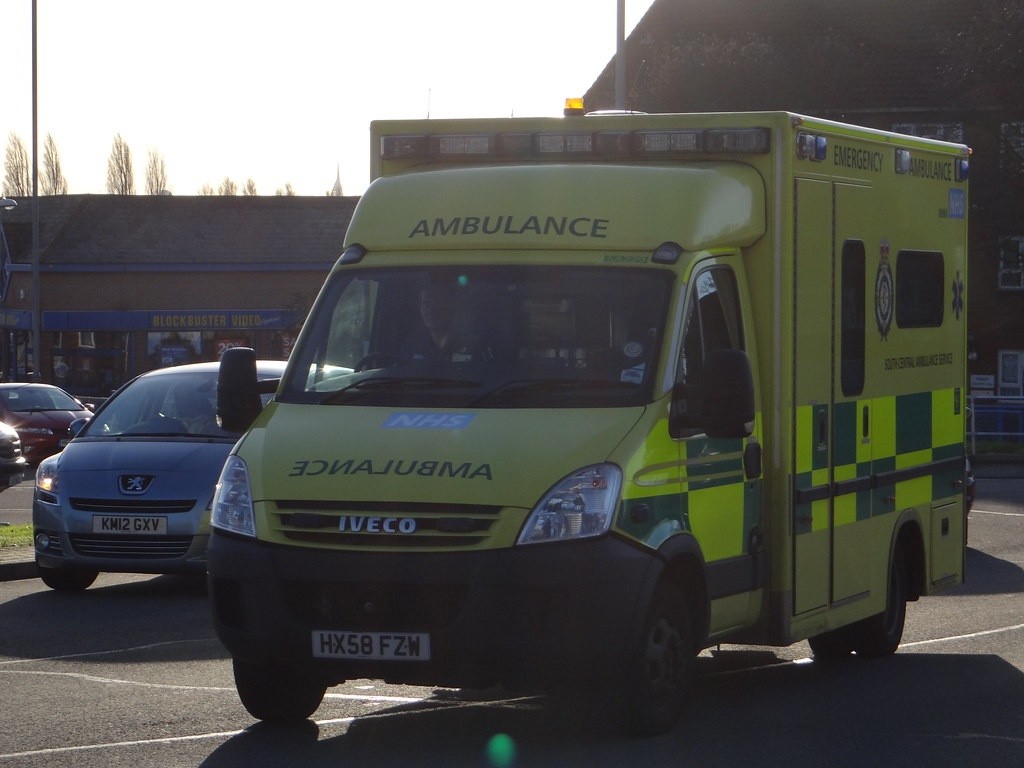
[202,110,974,734]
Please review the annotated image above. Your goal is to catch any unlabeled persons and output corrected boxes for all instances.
[54,360,70,386]
[395,280,482,378]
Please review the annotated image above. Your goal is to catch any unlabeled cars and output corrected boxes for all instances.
[0,382,99,491]
[32,361,356,595]
